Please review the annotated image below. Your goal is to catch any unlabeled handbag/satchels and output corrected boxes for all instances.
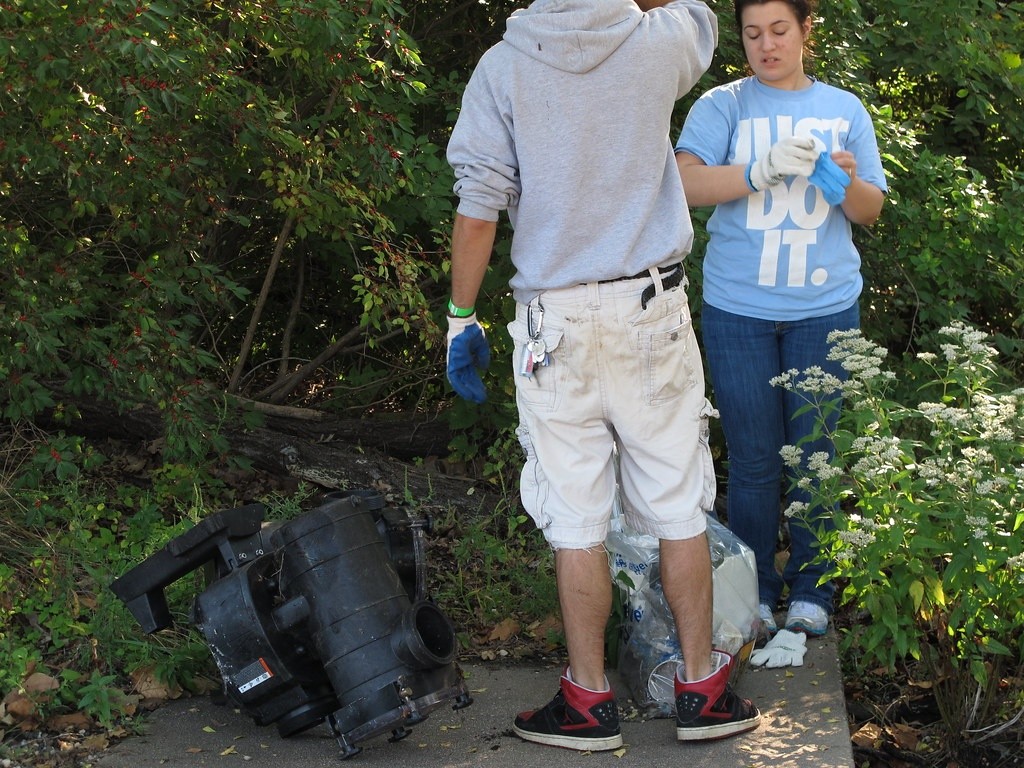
[604,483,760,721]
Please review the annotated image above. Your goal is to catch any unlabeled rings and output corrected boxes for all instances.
[849,167,852,177]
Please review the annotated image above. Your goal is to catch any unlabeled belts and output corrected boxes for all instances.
[580,262,684,310]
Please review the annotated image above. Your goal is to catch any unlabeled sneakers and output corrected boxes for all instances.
[512,663,623,751]
[674,649,762,740]
[760,604,778,633]
[785,601,829,636]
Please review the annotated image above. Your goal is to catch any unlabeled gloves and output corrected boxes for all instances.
[750,628,807,669]
[745,136,818,192]
[446,309,490,403]
[807,150,851,206]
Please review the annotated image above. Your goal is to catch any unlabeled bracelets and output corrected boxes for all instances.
[447,297,475,317]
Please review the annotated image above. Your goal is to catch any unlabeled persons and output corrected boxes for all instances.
[672,0,888,636]
[446,0,761,752]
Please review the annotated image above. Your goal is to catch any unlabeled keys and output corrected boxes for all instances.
[518,339,549,388]
